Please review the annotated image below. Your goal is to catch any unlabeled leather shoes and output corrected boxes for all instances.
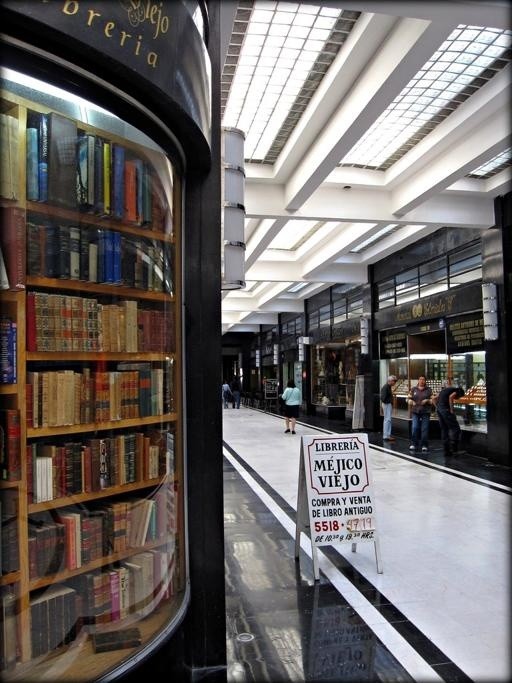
[388,435,397,440]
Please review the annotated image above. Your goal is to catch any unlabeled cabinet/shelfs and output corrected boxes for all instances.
[1,90,185,682]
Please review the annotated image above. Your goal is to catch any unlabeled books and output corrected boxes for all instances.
[28,223,179,656]
[1,112,178,238]
[1,207,21,672]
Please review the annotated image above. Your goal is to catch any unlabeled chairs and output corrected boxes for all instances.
[241,390,276,413]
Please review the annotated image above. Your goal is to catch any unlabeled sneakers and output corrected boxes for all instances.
[422,446,428,451]
[409,445,415,450]
[284,429,296,434]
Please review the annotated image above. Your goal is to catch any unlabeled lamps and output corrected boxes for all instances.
[255,350,260,368]
[219,125,247,292]
[298,336,304,361]
[273,343,279,365]
[481,283,499,341]
[360,318,369,354]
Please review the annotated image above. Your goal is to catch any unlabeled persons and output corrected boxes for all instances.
[222,375,242,409]
[405,375,434,451]
[432,387,467,454]
[380,374,398,441]
[279,378,302,434]
